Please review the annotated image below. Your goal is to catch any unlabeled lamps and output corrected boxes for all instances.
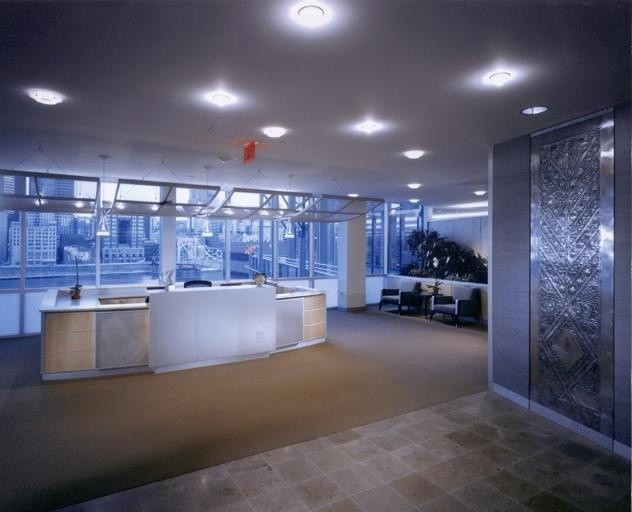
[285,174,294,239]
[201,166,213,236]
[95,156,113,236]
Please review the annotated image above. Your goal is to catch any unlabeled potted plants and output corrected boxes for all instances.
[68,283,82,299]
[427,281,443,294]
[161,269,176,292]
[251,271,266,288]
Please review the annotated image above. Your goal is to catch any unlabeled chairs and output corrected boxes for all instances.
[428,287,482,328]
[378,280,421,313]
[184,280,212,288]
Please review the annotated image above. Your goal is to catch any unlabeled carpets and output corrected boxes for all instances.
[0,309,489,512]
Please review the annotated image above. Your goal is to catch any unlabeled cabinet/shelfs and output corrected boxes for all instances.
[100,298,147,304]
